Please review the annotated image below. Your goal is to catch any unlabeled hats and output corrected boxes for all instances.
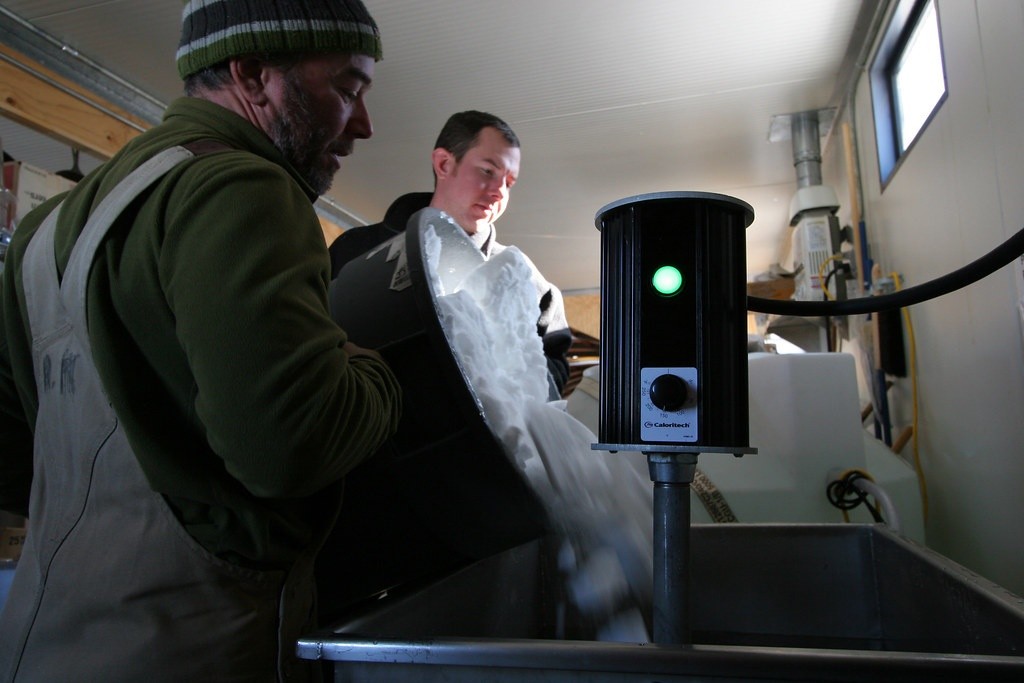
[175,0,383,80]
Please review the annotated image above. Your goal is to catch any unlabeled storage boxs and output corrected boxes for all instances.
[4,161,78,231]
[0,511,30,560]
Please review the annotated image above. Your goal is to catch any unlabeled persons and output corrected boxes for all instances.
[328,110,572,393]
[0,0,404,683]
